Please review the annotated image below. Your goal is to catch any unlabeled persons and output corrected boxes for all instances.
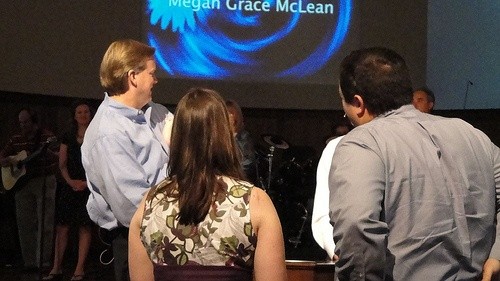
[311,135,358,281]
[224,98,255,181]
[413,88,435,113]
[42,100,96,281]
[0,107,58,281]
[330,46,500,281]
[80,38,174,281]
[128,86,286,281]
[325,123,355,141]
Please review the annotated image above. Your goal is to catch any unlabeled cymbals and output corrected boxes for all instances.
[261,135,291,150]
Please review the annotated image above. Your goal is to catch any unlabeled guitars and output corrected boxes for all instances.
[1,136,57,190]
